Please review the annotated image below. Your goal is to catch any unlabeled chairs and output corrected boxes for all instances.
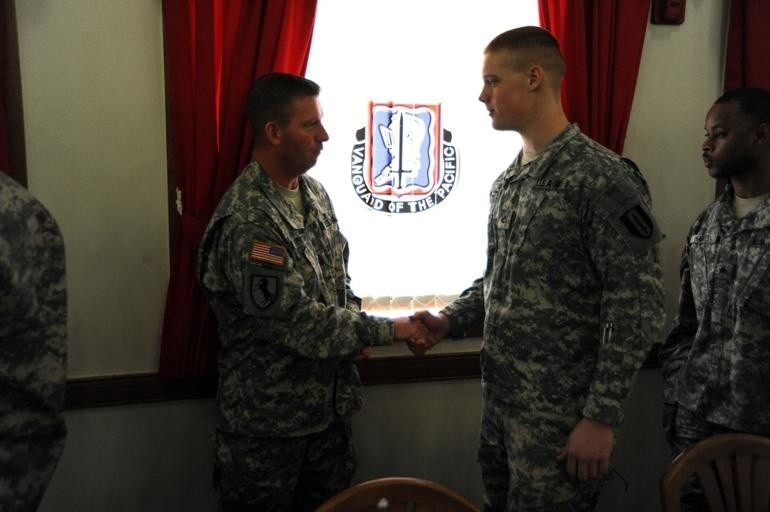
[318,477,479,512]
[662,431,770,511]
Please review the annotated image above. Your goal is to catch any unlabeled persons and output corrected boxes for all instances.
[195,70,435,512]
[659,88,770,512]
[0,166,72,512]
[404,22,670,512]
